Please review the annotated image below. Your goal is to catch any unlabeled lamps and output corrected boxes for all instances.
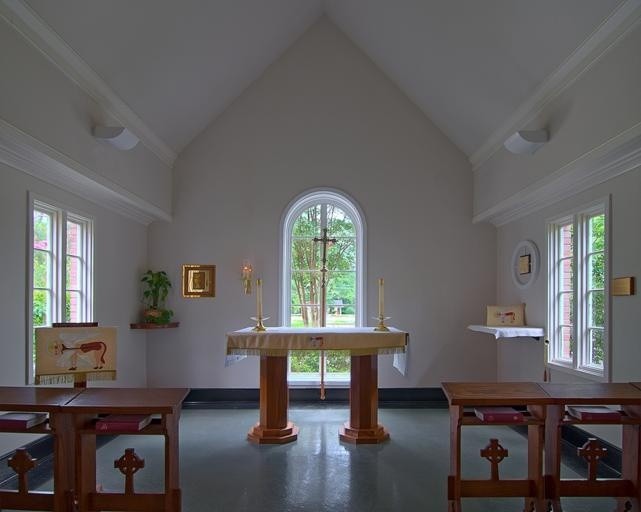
[94,125,140,152]
[504,130,550,156]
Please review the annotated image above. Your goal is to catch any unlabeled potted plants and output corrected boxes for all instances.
[140,268,174,324]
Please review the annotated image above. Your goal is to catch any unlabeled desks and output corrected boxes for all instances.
[439,378,641,510]
[227,326,408,445]
[1,385,191,512]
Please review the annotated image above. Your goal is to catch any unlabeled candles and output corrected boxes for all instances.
[379,278,386,319]
[257,279,263,320]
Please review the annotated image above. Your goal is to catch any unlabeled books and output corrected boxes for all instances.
[0,413,48,429]
[567,406,622,422]
[474,407,523,423]
[95,414,152,432]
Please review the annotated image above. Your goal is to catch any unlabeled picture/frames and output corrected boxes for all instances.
[182,264,217,298]
